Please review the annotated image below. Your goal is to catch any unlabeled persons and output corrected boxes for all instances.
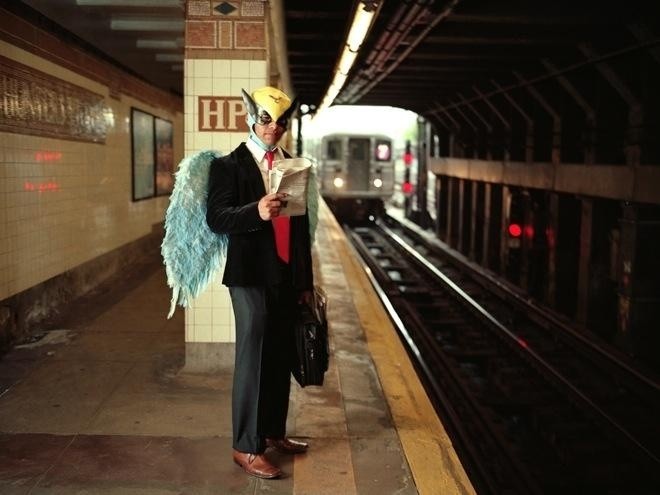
[206,86,312,478]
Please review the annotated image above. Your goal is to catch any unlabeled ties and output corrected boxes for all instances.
[264,151,274,170]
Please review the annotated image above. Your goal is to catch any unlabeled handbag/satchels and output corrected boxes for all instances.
[291,302,329,388]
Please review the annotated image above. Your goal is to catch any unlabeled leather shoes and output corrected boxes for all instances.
[232,438,308,478]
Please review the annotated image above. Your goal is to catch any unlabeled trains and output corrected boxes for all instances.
[317,133,395,224]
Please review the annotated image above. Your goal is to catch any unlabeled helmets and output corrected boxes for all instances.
[242,87,297,131]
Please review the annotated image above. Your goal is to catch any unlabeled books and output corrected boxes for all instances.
[269,157,312,216]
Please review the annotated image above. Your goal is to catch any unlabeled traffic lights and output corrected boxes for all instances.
[402,153,412,194]
